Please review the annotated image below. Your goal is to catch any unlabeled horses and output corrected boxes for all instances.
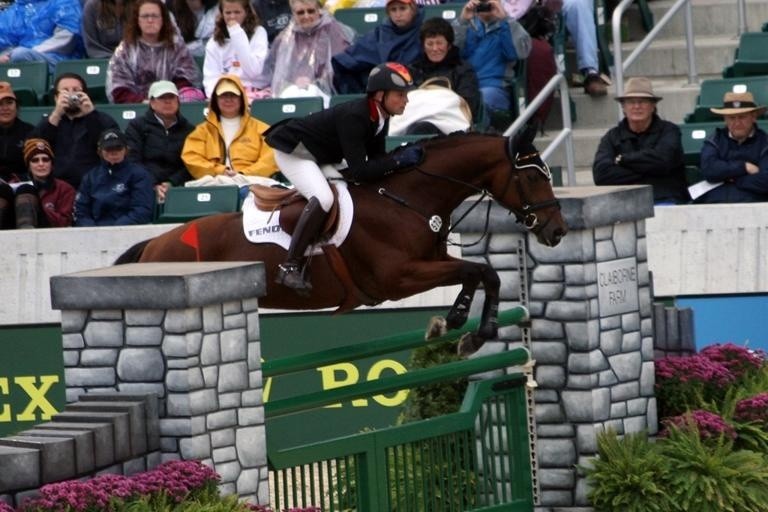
[112,117,568,357]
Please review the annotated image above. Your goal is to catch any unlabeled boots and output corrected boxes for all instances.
[275,196,329,299]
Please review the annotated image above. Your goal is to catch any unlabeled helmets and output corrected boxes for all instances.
[366,61,417,94]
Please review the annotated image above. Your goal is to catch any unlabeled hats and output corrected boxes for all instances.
[615,77,662,101]
[709,92,767,114]
[0,78,242,161]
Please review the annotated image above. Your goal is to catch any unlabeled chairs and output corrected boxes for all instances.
[0,0,469,223]
[671,18,767,186]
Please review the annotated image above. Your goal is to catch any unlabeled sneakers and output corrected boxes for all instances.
[583,74,606,98]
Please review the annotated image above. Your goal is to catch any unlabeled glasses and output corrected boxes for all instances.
[294,9,313,14]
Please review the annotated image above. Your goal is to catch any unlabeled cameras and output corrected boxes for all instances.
[66,93,81,105]
[476,3,490,11]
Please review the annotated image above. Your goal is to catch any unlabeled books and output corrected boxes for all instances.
[684,178,729,200]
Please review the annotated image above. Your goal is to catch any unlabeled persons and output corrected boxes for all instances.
[0,0,611,229]
[685,91,767,204]
[592,76,687,204]
[261,61,428,297]
